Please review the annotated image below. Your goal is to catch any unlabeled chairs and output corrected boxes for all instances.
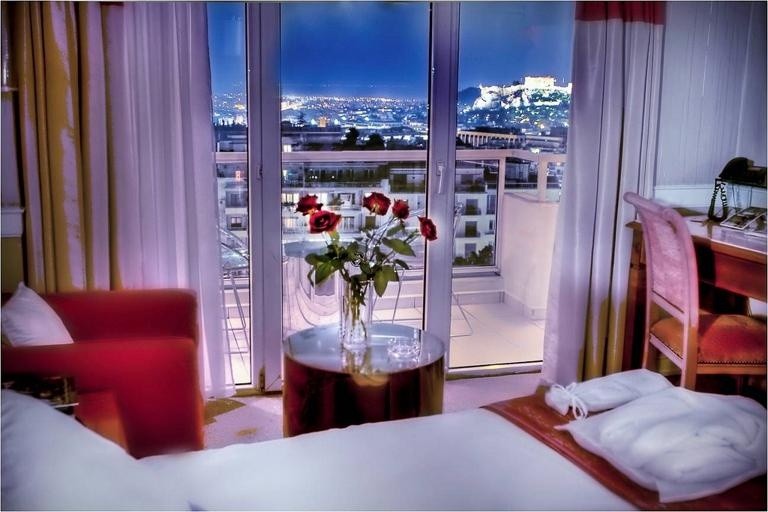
[2,287,204,456]
[622,193,766,391]
[372,199,473,335]
[219,222,290,386]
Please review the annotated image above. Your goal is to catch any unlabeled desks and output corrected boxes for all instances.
[627,215,766,394]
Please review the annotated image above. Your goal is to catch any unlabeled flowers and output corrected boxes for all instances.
[293,191,437,320]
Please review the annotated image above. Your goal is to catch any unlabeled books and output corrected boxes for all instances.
[742,213,767,239]
[719,206,767,230]
[1,374,81,429]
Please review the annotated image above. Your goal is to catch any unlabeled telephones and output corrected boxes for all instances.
[718,157,767,189]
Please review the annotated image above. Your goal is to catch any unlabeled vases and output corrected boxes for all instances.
[340,282,368,347]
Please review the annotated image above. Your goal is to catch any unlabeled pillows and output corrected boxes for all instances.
[1,280,75,348]
[1,390,191,512]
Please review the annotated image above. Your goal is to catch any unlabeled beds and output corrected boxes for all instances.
[136,368,766,512]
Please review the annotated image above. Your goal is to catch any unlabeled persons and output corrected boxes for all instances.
[753,217,765,230]
